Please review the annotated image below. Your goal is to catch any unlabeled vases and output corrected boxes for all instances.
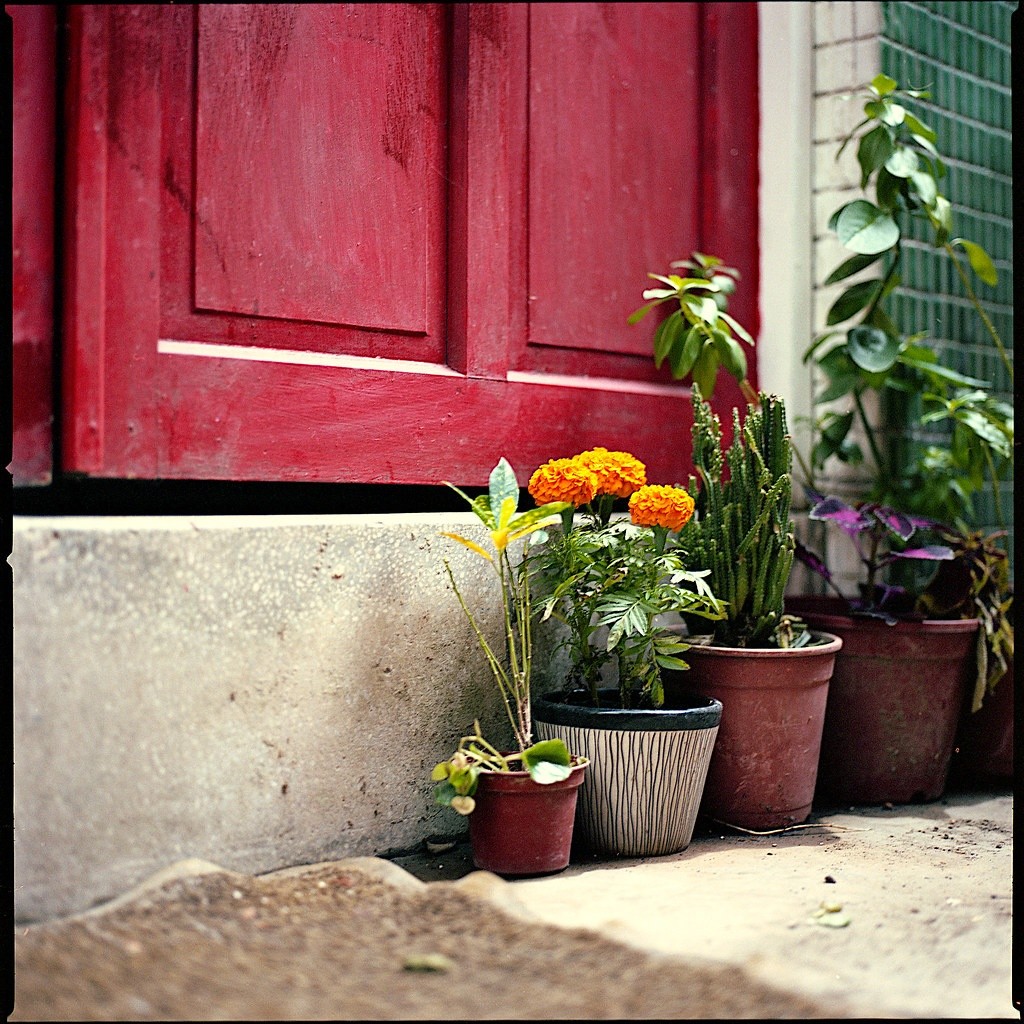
[531,703,721,860]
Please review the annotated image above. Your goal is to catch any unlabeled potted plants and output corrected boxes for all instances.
[679,388,844,839]
[631,80,983,806]
[430,459,592,877]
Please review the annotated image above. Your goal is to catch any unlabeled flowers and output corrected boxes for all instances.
[529,448,726,710]
